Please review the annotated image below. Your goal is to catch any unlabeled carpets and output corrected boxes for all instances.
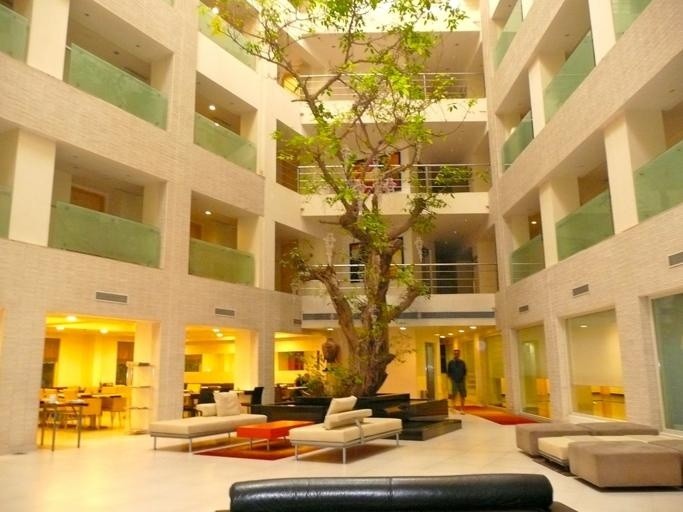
[193,438,320,460]
[452,404,549,425]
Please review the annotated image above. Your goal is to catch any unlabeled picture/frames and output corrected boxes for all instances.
[350,151,402,195]
[349,236,404,284]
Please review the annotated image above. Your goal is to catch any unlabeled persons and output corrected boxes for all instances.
[298,373,312,396]
[446,348,467,415]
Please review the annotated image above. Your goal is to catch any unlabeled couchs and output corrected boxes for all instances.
[215,474,577,512]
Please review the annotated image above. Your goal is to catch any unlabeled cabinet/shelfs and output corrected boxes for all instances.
[129,363,153,410]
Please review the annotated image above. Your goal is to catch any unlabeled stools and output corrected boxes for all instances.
[578,421,660,436]
[516,422,591,458]
[568,440,680,490]
[648,438,683,488]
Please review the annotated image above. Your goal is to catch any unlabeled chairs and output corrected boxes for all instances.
[183,382,307,416]
[36,383,130,451]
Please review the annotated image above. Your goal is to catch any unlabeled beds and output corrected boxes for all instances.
[149,402,268,452]
[288,416,403,465]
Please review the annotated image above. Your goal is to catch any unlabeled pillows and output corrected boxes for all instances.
[322,395,357,428]
[324,408,374,430]
